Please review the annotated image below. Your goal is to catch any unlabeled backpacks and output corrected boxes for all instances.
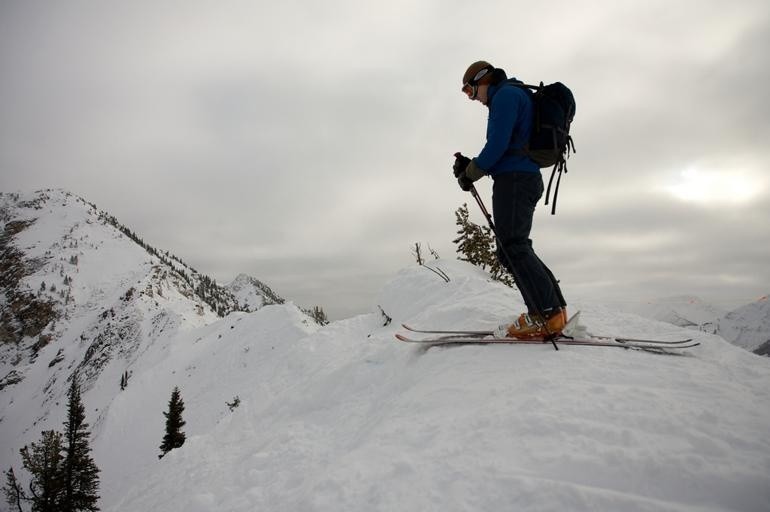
[487,78,576,165]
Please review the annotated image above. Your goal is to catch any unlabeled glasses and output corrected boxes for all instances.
[462,65,490,100]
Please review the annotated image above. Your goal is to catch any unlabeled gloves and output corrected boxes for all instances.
[452,155,487,191]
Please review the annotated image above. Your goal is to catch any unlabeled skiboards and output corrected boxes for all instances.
[394,321,700,350]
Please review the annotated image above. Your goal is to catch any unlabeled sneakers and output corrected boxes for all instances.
[507,303,569,339]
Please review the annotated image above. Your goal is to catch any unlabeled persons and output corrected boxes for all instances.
[452,61,568,339]
